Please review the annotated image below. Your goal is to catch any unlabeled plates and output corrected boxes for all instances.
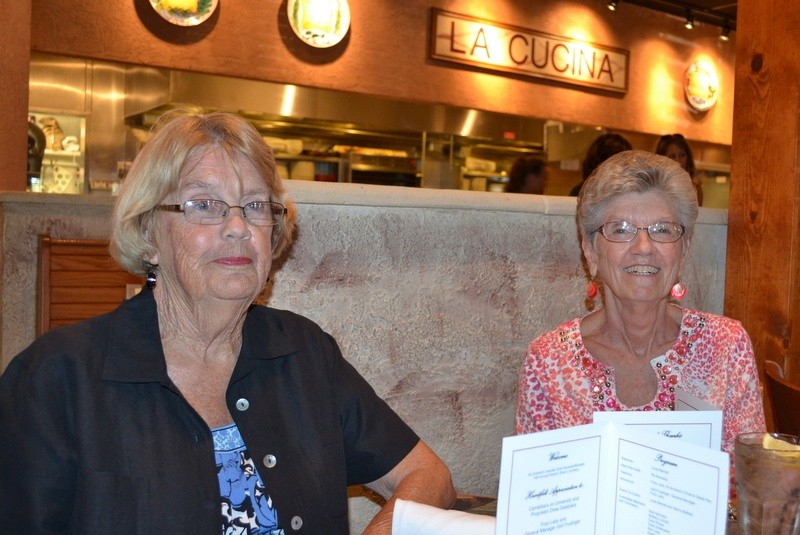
[287,0,350,48]
[685,57,718,110]
[149,0,218,26]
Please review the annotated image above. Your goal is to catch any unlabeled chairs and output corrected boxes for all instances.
[34,234,144,336]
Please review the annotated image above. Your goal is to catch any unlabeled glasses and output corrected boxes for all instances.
[149,198,290,226]
[589,220,685,243]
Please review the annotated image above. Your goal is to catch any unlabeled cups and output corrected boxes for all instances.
[734,431,800,535]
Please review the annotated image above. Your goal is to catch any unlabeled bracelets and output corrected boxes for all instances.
[727,501,738,521]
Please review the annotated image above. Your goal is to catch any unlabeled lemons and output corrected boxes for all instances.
[762,433,800,468]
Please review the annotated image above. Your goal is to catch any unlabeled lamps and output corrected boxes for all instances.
[607,0,619,12]
[720,25,731,42]
[684,14,696,30]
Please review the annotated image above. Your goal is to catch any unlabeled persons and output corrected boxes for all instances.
[514,151,770,522]
[504,133,703,209]
[0,113,457,535]
